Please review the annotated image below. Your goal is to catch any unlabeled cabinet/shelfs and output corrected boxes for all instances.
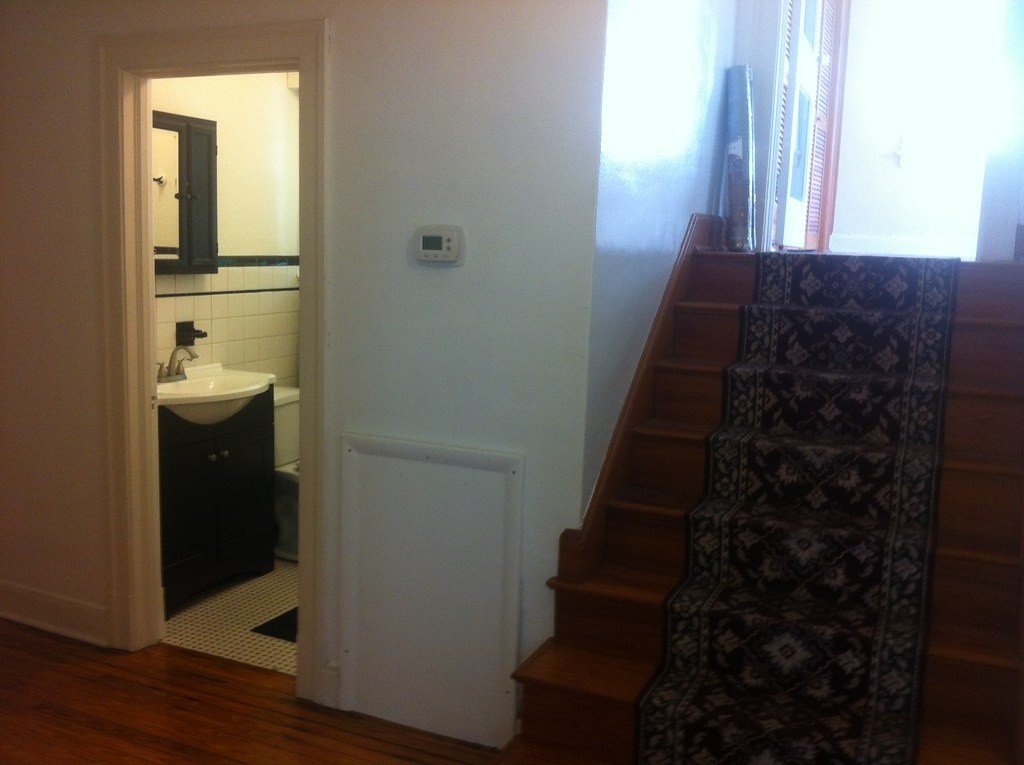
[158,383,275,623]
[152,110,218,275]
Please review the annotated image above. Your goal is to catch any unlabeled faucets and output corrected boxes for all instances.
[167,345,199,383]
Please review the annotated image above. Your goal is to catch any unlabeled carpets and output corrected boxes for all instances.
[249,606,297,643]
[630,251,960,765]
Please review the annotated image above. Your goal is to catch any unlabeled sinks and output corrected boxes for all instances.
[157,362,277,425]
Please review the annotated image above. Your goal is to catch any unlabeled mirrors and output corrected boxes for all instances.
[153,126,180,259]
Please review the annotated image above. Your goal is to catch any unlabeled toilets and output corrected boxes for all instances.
[273,384,300,563]
